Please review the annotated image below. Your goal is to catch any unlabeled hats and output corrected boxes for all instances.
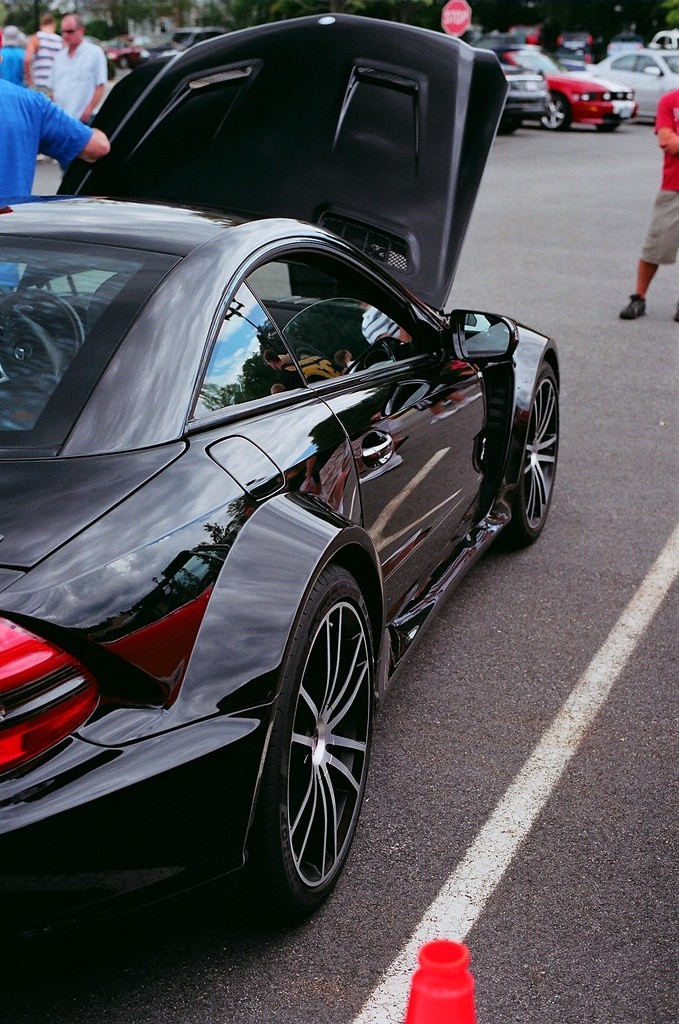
[2,25,26,46]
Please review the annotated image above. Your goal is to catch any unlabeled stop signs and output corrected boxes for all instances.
[441,0,471,37]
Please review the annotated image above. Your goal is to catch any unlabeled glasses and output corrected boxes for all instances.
[62,27,79,33]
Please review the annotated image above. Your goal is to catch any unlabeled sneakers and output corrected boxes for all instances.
[673,301,679,321]
[619,293,645,320]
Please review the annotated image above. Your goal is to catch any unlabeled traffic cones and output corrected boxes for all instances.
[402,939,479,1024]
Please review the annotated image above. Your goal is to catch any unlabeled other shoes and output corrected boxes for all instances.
[36,153,58,165]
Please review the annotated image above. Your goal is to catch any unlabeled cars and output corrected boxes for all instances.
[584,49,679,118]
[558,31,604,55]
[647,29,679,49]
[606,32,644,57]
[104,35,151,69]
[139,27,232,66]
[0,13,560,949]
[470,25,639,133]
[496,58,551,136]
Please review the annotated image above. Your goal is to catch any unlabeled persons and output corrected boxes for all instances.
[619,86,679,323]
[0,13,111,196]
[239,299,483,518]
